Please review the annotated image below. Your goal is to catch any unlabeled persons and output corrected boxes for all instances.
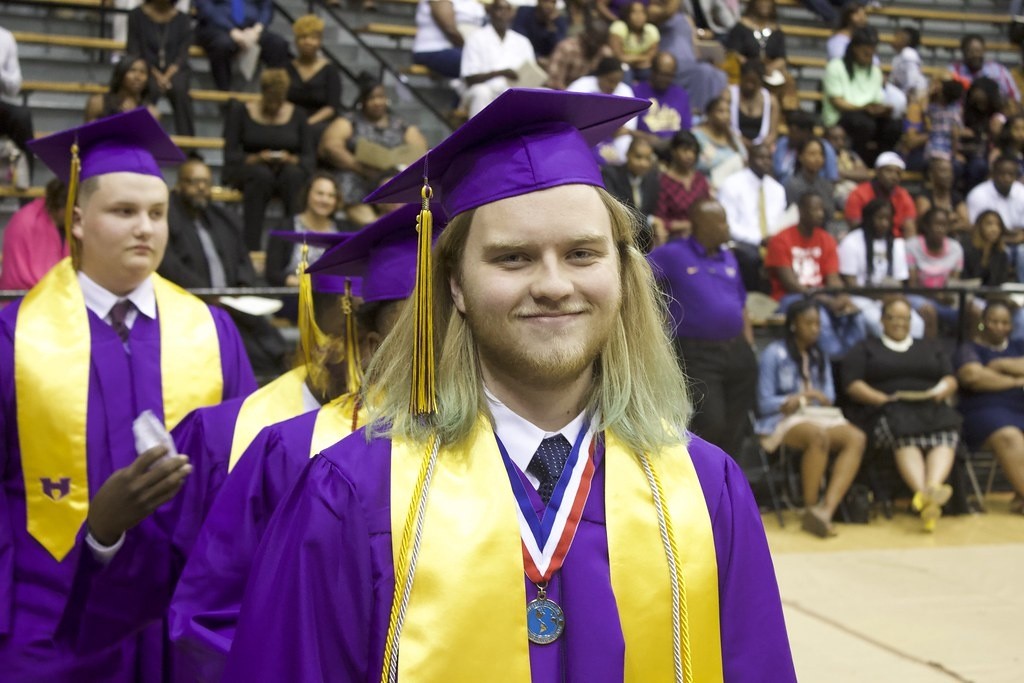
[411,0,1024,541]
[0,0,434,683]
[224,89,797,683]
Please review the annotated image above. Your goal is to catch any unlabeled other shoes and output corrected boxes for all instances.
[802,506,837,538]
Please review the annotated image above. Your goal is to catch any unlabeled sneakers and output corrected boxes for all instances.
[921,505,941,531]
[913,485,952,512]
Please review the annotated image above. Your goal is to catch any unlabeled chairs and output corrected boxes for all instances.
[730,374,1024,538]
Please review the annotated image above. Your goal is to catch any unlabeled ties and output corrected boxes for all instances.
[526,432,572,506]
[193,217,227,288]
[109,301,130,344]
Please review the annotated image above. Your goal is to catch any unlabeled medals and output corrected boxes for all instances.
[527,597,565,644]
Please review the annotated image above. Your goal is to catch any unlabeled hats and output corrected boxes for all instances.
[874,151,907,169]
[304,204,448,419]
[854,25,877,44]
[25,107,188,272]
[362,88,653,416]
[270,231,358,398]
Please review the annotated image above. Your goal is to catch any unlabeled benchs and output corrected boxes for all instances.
[0,0,328,281]
[345,0,1024,279]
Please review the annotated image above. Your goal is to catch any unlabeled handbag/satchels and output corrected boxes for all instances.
[883,396,964,436]
